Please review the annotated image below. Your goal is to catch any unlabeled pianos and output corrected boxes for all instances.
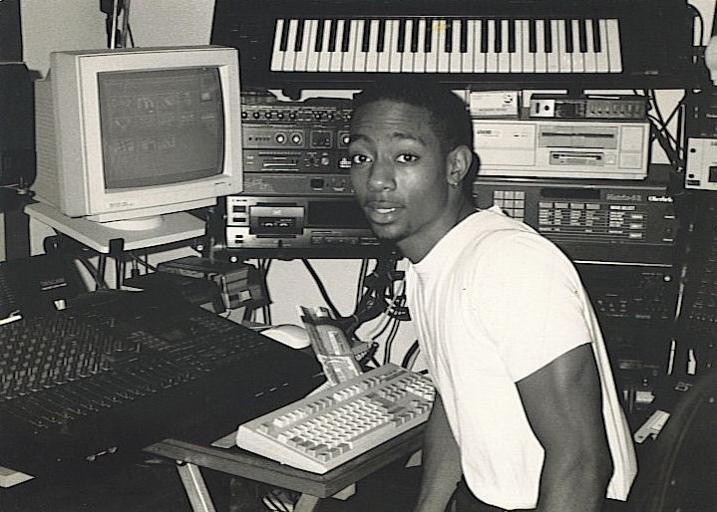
[208,0,710,91]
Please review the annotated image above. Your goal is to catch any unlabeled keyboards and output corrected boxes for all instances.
[236,364,435,476]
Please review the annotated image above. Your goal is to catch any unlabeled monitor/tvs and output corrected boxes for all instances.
[35,44,243,252]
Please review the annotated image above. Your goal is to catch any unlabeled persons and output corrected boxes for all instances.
[349,77,637,512]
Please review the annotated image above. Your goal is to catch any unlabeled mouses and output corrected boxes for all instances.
[261,324,310,350]
